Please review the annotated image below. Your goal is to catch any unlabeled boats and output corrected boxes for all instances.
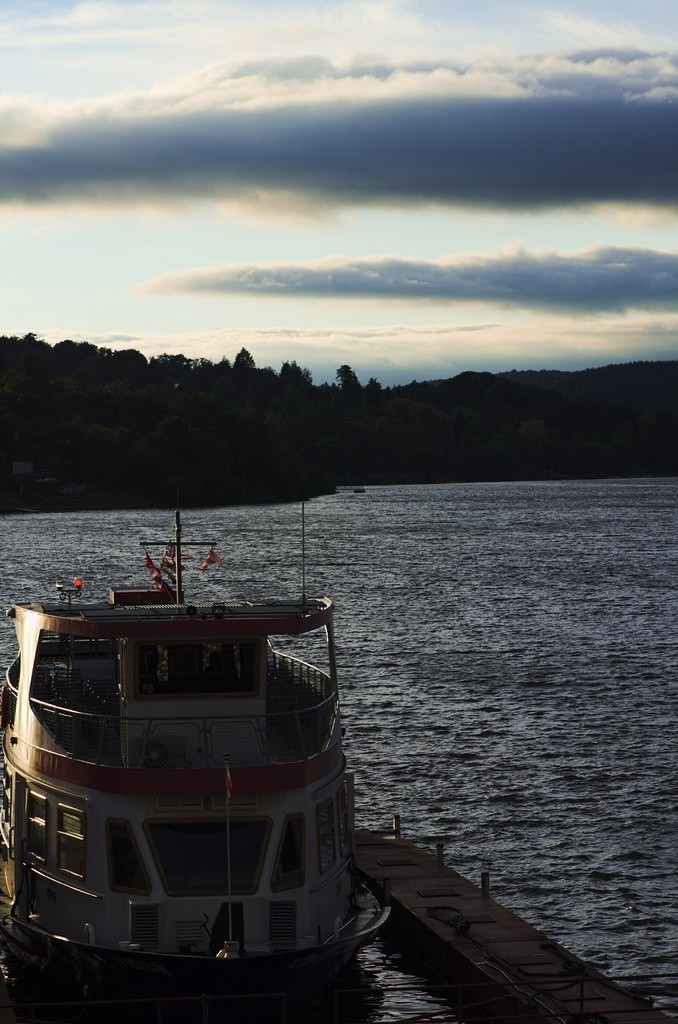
[0,510,393,1015]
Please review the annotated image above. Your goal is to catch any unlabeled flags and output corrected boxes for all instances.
[199,550,220,569]
[226,768,233,802]
[146,545,194,583]
